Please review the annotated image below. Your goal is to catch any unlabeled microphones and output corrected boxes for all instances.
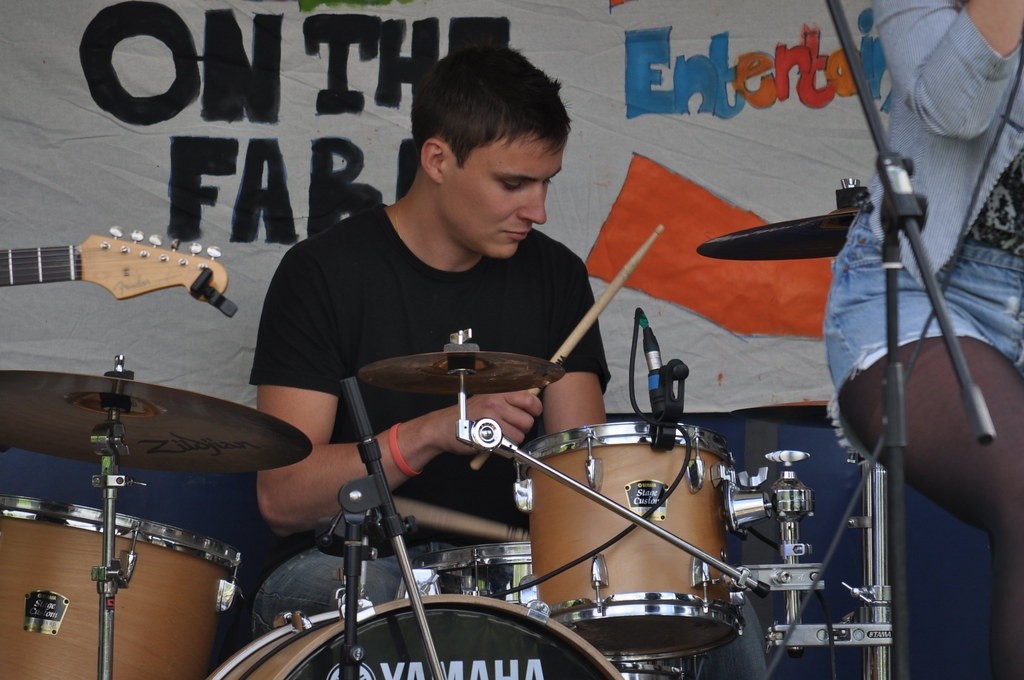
[638,310,676,417]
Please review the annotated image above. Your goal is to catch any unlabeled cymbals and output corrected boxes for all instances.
[697,205,861,260]
[356,351,566,394]
[0,368,314,477]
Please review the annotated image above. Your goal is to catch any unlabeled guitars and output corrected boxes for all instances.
[0,224,239,318]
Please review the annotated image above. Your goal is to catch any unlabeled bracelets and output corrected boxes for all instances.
[389,422,422,477]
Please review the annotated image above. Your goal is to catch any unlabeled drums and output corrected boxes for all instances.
[0,492,241,680]
[411,540,541,616]
[202,594,623,680]
[522,419,742,657]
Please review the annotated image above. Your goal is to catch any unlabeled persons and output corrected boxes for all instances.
[823,0,1024,680]
[249,48,611,642]
